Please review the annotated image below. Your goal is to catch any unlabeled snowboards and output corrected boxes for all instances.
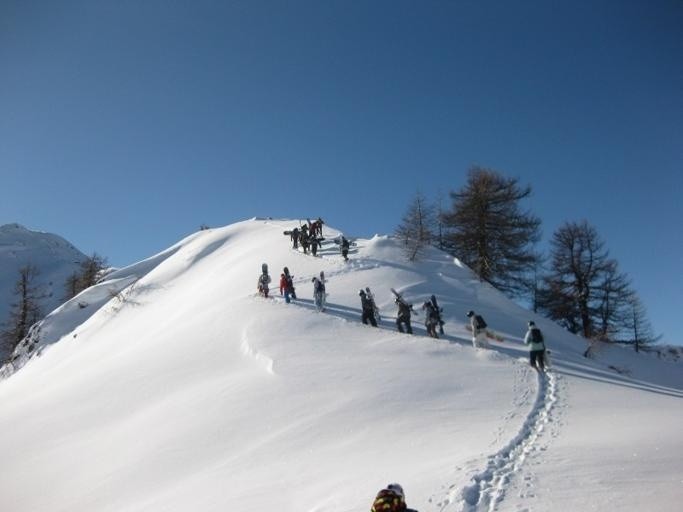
[306,217,312,226]
[284,231,293,235]
[334,240,357,246]
[543,348,553,368]
[365,287,381,320]
[284,267,296,299]
[464,325,505,342]
[431,295,444,335]
[263,263,268,295]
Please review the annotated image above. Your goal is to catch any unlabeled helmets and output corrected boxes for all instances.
[388,484,403,501]
[527,321,534,326]
[466,311,474,317]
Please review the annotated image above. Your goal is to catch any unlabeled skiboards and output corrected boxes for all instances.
[320,271,325,305]
[390,288,418,316]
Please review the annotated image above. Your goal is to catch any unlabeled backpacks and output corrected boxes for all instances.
[476,315,487,329]
[371,490,406,512]
[530,328,542,343]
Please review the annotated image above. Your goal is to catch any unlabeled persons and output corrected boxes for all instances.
[256,273,270,298]
[394,298,412,335]
[421,300,438,338]
[358,289,377,327]
[311,277,323,312]
[466,310,488,349]
[290,216,325,257]
[338,236,349,261]
[369,484,417,512]
[278,274,295,305]
[522,320,545,372]
